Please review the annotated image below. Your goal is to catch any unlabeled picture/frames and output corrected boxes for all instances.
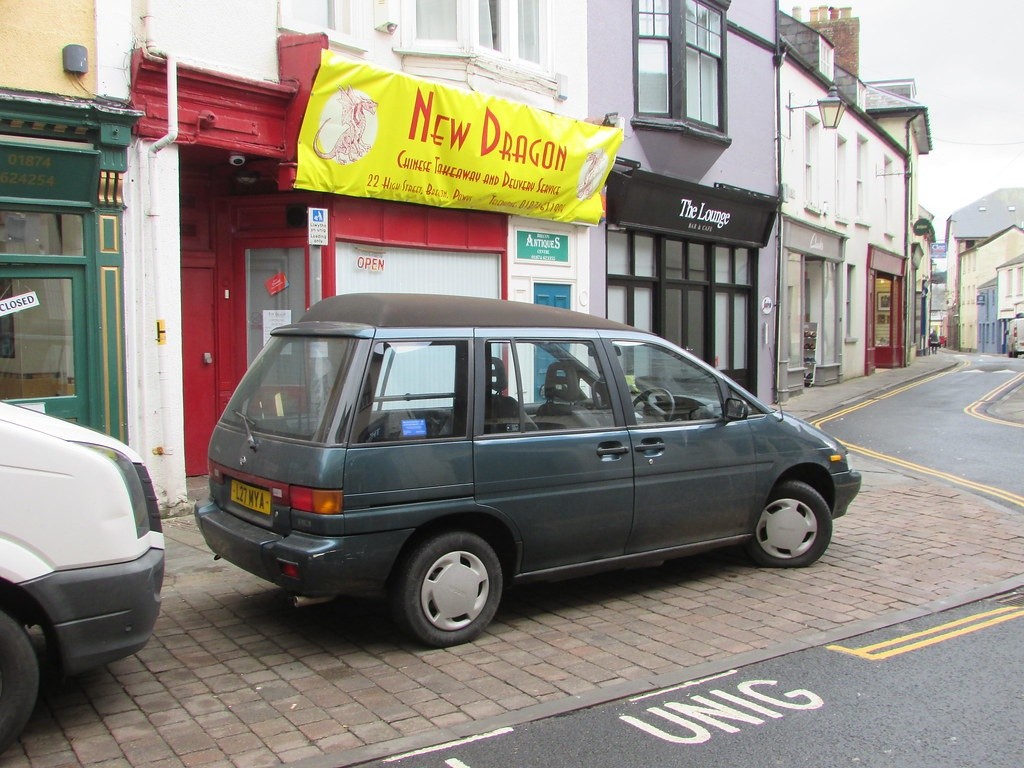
[877,291,891,311]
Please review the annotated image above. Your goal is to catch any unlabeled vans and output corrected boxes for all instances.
[1004,319,1024,358]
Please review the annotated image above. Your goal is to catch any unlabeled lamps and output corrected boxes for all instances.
[790,79,850,130]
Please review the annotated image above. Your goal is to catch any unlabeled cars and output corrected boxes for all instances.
[193,294,863,647]
[0,401,168,757]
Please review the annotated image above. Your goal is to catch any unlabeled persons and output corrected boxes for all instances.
[929,330,940,355]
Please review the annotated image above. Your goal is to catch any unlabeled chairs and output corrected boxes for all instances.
[531,361,600,430]
[491,357,540,432]
